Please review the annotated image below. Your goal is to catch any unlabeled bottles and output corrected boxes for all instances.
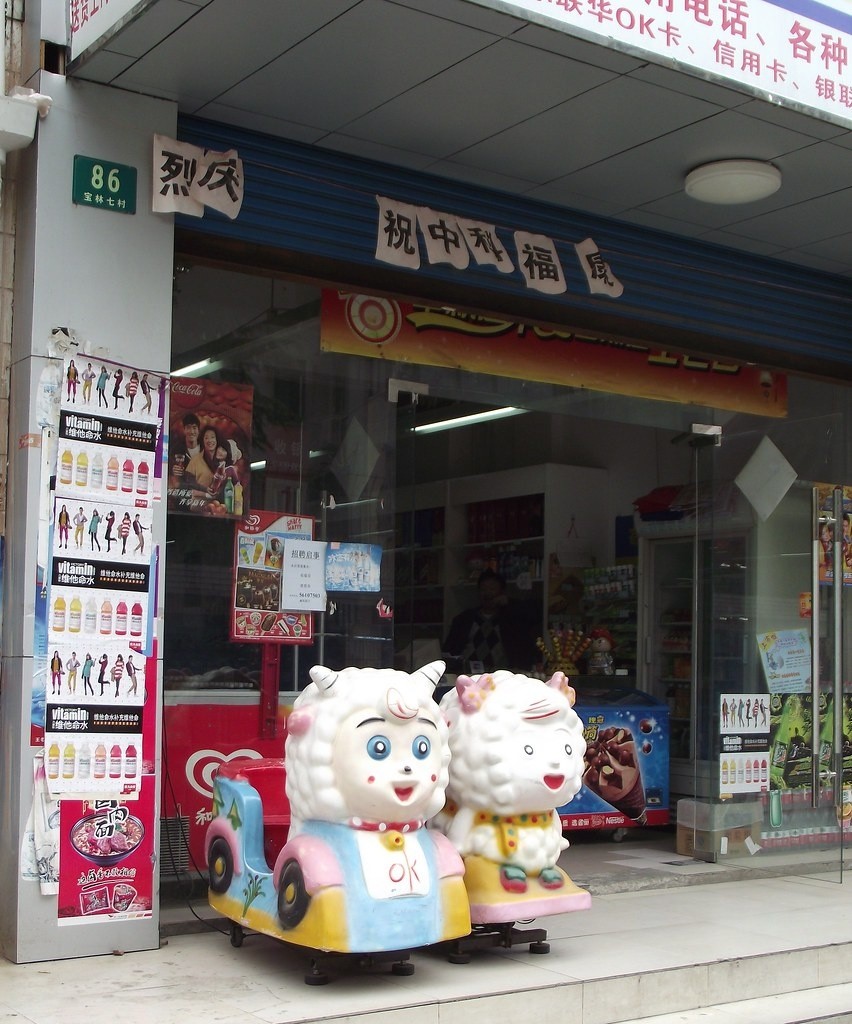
[61,448,149,494]
[235,481,243,515]
[660,633,693,720]
[94,743,106,778]
[53,596,143,636]
[224,477,234,513]
[49,743,59,779]
[723,758,769,782]
[63,742,75,778]
[212,461,226,491]
[125,743,137,778]
[756,788,852,849]
[79,743,90,778]
[581,565,633,594]
[109,744,122,778]
[498,546,529,576]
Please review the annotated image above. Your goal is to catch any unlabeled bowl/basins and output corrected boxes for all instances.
[71,813,145,867]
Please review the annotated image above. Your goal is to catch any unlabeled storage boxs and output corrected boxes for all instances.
[396,638,440,670]
[676,798,762,862]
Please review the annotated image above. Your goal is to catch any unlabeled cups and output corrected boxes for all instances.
[174,455,184,476]
[293,624,302,636]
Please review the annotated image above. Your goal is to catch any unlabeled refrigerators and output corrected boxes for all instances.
[634,509,753,799]
[159,691,299,882]
[556,686,669,828]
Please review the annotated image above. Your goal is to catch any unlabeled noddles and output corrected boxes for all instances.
[72,817,142,856]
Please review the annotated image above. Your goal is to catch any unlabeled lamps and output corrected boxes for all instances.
[684,160,784,206]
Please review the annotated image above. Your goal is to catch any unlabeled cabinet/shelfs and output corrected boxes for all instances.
[322,463,636,675]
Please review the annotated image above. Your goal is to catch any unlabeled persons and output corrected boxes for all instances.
[58,505,148,555]
[67,360,157,414]
[51,651,141,697]
[168,414,239,510]
[438,570,533,674]
[819,514,852,562]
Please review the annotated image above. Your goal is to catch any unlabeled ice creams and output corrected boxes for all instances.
[581,727,648,826]
